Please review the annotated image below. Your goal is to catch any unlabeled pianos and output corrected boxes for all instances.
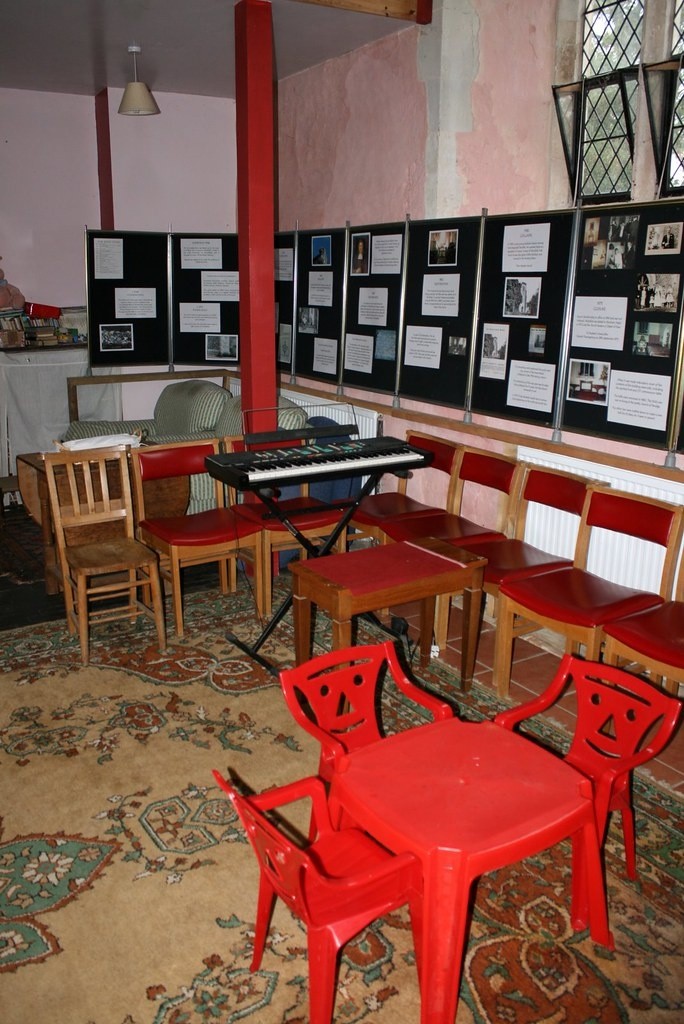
[204,402,434,680]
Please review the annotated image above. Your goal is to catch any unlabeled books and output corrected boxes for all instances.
[0,308,79,347]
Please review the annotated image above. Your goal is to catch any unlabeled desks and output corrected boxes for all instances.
[313,716,614,1024]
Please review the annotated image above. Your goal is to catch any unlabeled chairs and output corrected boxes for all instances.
[494,653,681,929]
[46,444,168,668]
[211,767,426,1023]
[603,535,684,716]
[274,642,457,842]
[379,444,525,651]
[331,427,463,613]
[497,481,683,702]
[130,438,263,639]
[434,461,613,687]
[222,430,347,624]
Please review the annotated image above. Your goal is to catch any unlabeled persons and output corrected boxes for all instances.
[636,285,674,309]
[608,216,639,269]
[661,226,675,249]
[314,248,327,263]
[353,240,368,273]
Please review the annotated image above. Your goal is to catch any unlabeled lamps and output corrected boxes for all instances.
[117,44,161,117]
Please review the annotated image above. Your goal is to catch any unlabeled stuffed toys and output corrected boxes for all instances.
[0,256,26,309]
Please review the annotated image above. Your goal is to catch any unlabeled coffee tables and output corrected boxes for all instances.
[287,536,488,694]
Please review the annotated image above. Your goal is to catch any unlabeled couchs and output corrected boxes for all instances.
[63,380,307,514]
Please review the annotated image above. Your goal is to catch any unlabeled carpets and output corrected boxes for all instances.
[2,568,683,1023]
